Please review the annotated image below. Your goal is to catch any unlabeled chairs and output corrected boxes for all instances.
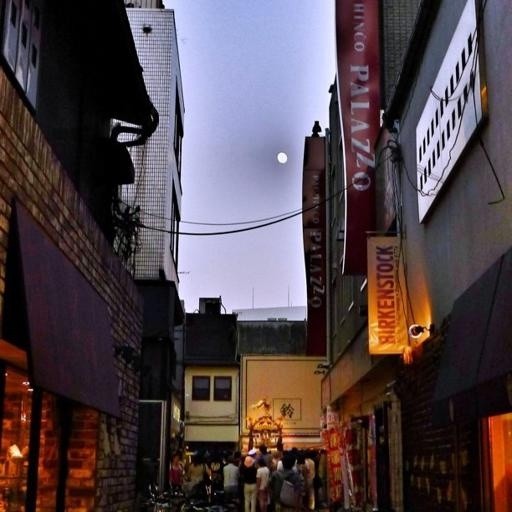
[0,455,94,512]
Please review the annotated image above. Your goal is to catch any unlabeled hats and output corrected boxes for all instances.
[243,456,254,468]
[247,448,257,456]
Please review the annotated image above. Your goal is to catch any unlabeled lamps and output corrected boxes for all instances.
[407,322,435,339]
[313,369,327,376]
[311,120,322,137]
[317,362,331,371]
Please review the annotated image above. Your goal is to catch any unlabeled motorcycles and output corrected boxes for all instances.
[145,484,239,512]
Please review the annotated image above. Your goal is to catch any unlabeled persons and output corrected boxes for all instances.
[159,442,328,512]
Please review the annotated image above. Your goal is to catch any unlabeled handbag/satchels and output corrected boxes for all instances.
[280,471,304,508]
